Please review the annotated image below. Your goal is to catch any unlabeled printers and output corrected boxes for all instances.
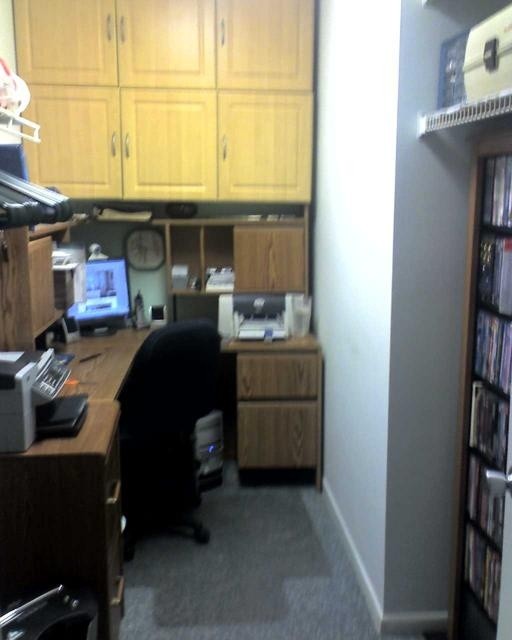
[218,293,312,340]
[0,349,89,452]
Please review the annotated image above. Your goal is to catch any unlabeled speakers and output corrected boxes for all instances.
[59,316,81,342]
[149,305,168,330]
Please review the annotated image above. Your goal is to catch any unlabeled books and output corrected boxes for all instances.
[462,157,511,624]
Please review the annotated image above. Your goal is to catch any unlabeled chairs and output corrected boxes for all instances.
[119,319,222,560]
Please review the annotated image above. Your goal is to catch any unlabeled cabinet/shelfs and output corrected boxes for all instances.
[217,1,314,204]
[162,218,305,292]
[449,137,512,638]
[0,218,77,351]
[11,1,218,202]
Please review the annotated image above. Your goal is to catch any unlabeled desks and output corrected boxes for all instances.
[0,329,323,639]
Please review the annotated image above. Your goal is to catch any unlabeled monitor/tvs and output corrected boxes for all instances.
[65,260,132,336]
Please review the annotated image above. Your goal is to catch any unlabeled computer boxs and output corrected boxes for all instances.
[195,410,223,491]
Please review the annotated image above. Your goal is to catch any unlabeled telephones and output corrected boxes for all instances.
[134,289,149,330]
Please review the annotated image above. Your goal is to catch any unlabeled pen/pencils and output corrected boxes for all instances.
[80,353,102,363]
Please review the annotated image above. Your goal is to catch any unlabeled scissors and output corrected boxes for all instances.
[65,377,92,386]
